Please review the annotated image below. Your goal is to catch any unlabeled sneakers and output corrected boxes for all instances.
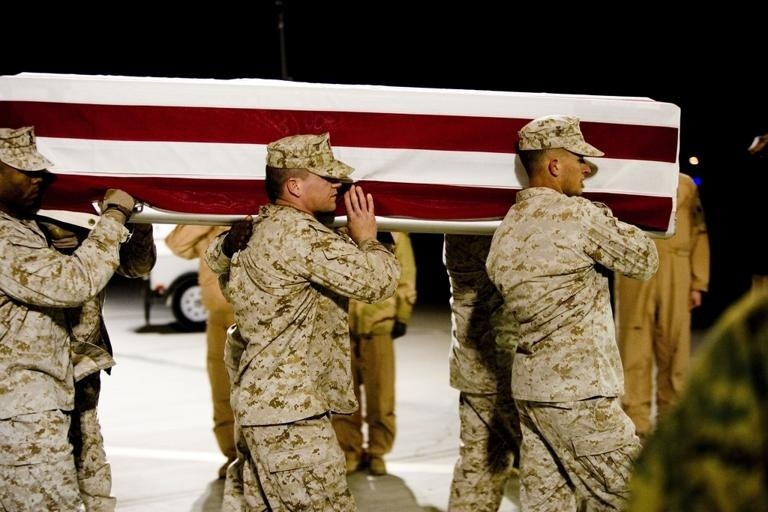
[220,456,232,479]
[345,458,387,477]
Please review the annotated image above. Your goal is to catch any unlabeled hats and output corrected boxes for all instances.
[265,131,355,179]
[517,116,604,158]
[0,127,54,173]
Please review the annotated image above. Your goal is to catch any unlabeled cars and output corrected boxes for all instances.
[148,222,206,327]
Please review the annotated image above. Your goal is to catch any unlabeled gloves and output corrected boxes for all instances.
[393,321,407,338]
[102,187,135,225]
